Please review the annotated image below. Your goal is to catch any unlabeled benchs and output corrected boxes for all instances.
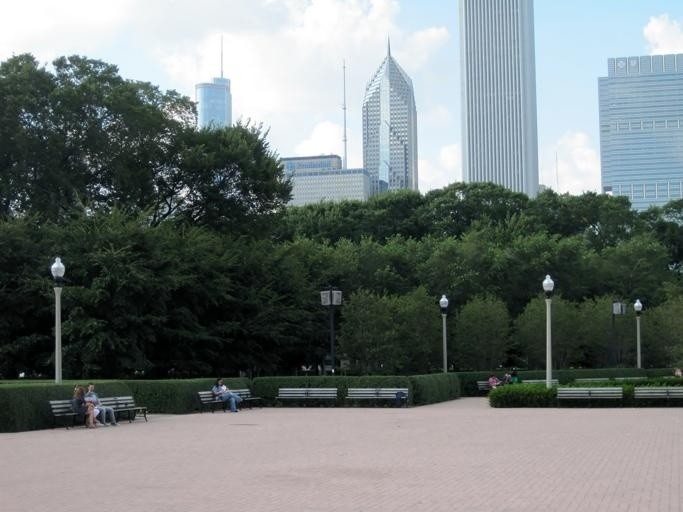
[196,383,410,412]
[47,394,148,429]
[476,375,683,406]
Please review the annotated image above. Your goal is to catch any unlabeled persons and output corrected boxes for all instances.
[213,377,246,414]
[73,387,99,430]
[85,384,118,428]
[502,370,519,384]
[488,375,501,388]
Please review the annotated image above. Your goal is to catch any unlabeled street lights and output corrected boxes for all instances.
[542,274,555,391]
[51,256,66,385]
[634,298,643,369]
[612,301,627,368]
[439,294,449,374]
[320,285,343,375]
